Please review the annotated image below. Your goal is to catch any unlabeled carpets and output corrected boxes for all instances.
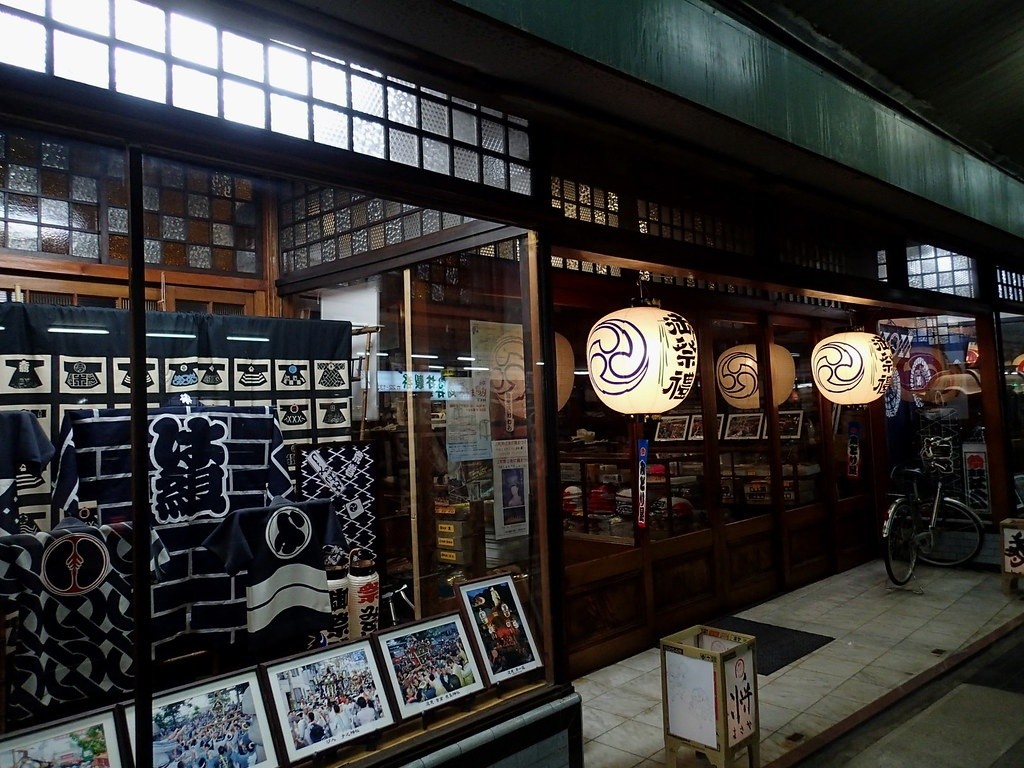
[654,615,836,675]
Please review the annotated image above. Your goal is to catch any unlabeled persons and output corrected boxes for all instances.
[153,617,533,768]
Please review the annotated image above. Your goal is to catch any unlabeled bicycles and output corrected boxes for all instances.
[881,454,986,596]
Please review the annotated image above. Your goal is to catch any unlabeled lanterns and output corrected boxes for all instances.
[488,327,575,419]
[716,344,796,410]
[587,297,699,426]
[349,560,380,640]
[894,346,946,403]
[811,331,893,406]
[326,565,349,644]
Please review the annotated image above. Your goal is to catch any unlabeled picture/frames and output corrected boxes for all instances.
[687,414,725,440]
[116,663,287,768]
[260,635,399,768]
[0,702,129,768]
[453,569,544,687]
[371,608,491,723]
[654,415,690,441]
[724,412,765,441]
[761,410,803,440]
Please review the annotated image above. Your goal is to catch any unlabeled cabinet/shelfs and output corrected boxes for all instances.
[558,378,827,540]
